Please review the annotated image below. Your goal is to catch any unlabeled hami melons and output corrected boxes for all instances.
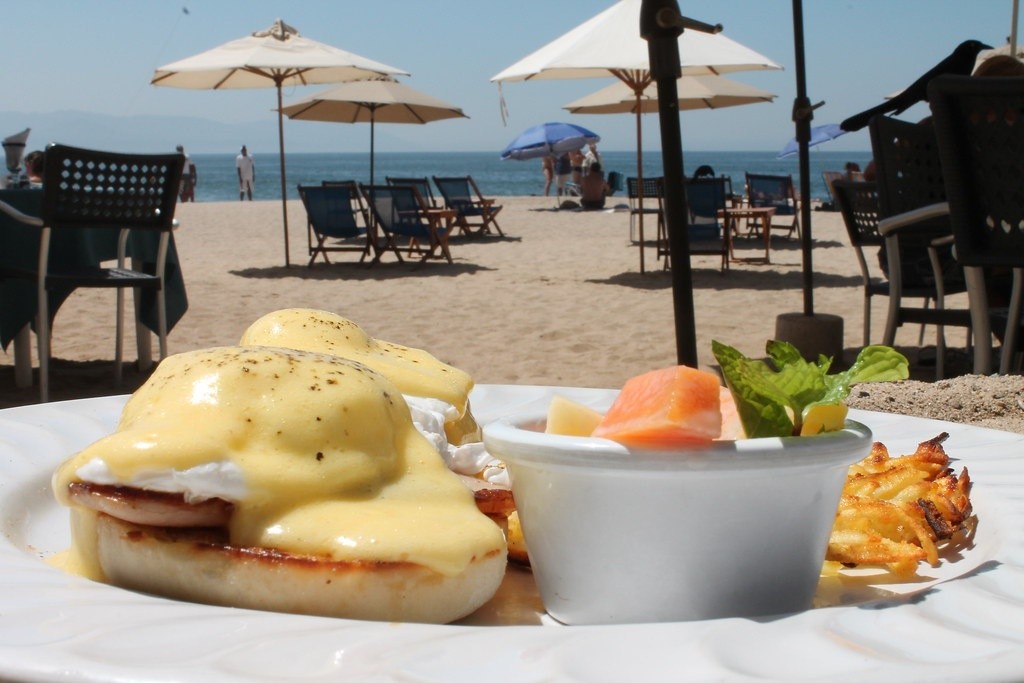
[591,364,742,439]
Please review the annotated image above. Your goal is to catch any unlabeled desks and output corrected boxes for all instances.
[714,208,775,265]
[397,209,458,257]
[0,189,189,389]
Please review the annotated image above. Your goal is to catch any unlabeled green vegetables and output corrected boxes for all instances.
[712,339,906,439]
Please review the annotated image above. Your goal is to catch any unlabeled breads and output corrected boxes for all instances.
[68,481,507,623]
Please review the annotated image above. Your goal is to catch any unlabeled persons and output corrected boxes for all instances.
[177,145,197,202]
[235,146,256,200]
[543,144,612,211]
[24,151,47,189]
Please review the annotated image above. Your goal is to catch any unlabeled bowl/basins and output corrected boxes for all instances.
[482,406,874,626]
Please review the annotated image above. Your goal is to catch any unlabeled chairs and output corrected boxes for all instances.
[820,70,1024,383]
[297,176,504,273]
[0,142,186,406]
[565,172,801,278]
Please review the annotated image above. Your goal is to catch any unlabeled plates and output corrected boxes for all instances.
[0,384,1024,683]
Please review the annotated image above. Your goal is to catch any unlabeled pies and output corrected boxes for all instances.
[822,432,970,582]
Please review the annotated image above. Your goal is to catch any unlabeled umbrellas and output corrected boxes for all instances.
[560,74,777,113]
[151,19,411,272]
[777,122,850,197]
[501,122,600,158]
[489,0,785,273]
[271,78,470,243]
